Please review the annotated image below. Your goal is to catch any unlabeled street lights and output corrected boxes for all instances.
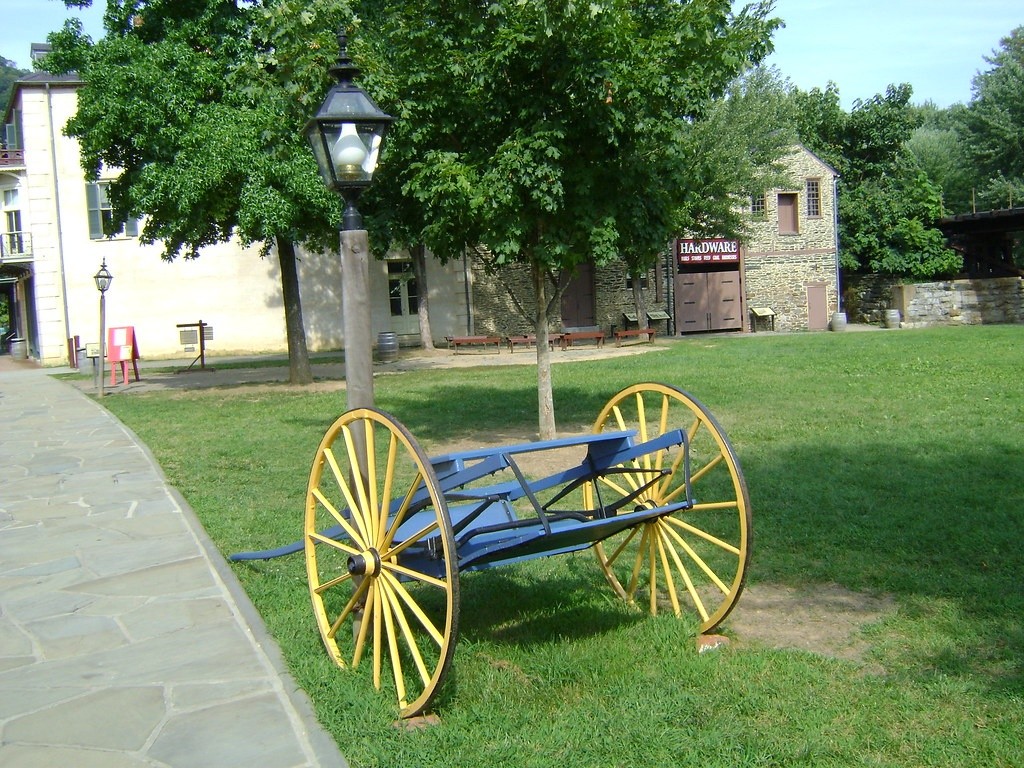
[298,32,399,647]
[92,256,113,399]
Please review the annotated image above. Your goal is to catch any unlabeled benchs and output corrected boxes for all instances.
[445,336,500,355]
[614,328,656,348]
[561,333,606,351]
[506,335,559,353]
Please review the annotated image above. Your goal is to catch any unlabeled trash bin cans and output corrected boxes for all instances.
[11,338,27,360]
[377,332,399,363]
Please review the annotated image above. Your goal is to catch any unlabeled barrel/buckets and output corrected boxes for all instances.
[831,313,847,332]
[377,332,398,362]
[885,310,900,328]
[77,348,100,375]
[11,338,27,359]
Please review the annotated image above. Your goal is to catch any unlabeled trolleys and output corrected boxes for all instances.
[221,379,756,723]
